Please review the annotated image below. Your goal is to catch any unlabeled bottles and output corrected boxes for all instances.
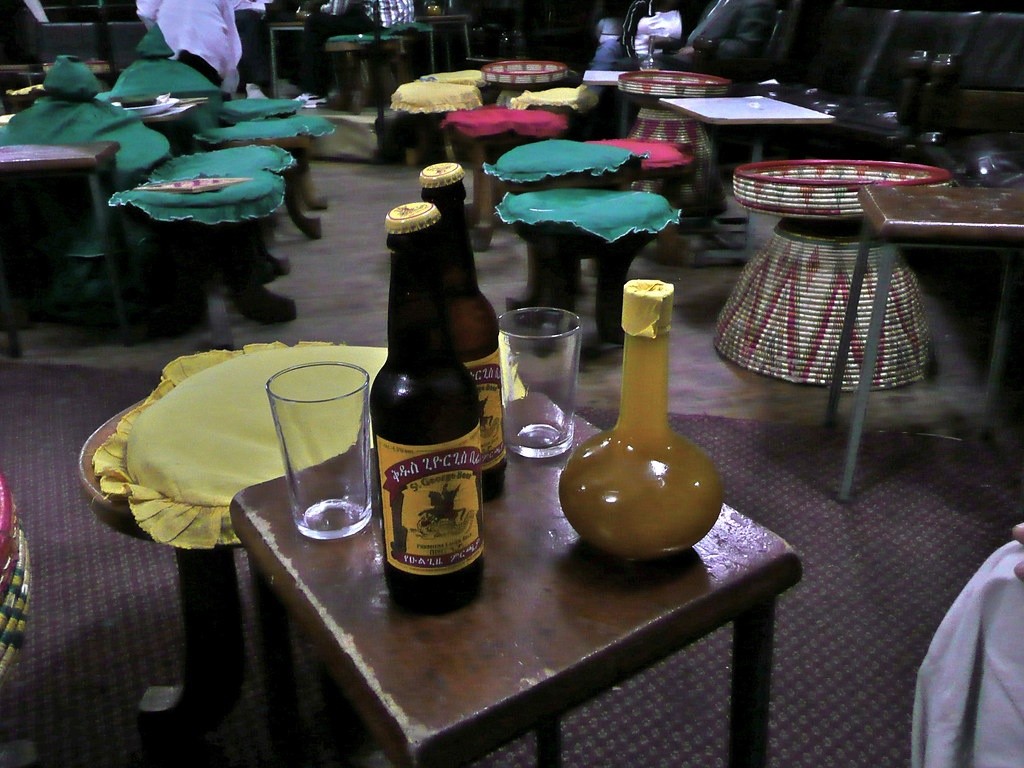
[559,278,723,562]
[420,161,507,498]
[370,201,484,614]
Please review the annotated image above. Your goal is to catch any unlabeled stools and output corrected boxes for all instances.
[585,139,696,180]
[440,108,569,252]
[325,23,433,115]
[480,140,652,328]
[78,342,527,670]
[390,82,483,166]
[413,70,491,87]
[492,188,683,345]
[497,84,600,113]
[5,84,338,351]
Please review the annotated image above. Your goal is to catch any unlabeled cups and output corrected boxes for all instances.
[497,305,581,460]
[266,360,371,540]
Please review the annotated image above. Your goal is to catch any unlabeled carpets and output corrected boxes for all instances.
[0,362,1024,768]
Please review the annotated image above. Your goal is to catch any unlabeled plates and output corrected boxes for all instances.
[111,97,180,117]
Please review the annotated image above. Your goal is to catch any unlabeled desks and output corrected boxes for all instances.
[829,186,1024,500]
[659,96,836,268]
[0,142,134,358]
[417,15,472,73]
[269,21,305,99]
[230,392,803,768]
[583,70,637,138]
[123,98,208,152]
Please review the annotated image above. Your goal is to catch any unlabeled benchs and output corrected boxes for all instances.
[38,22,147,81]
[692,0,1024,188]
